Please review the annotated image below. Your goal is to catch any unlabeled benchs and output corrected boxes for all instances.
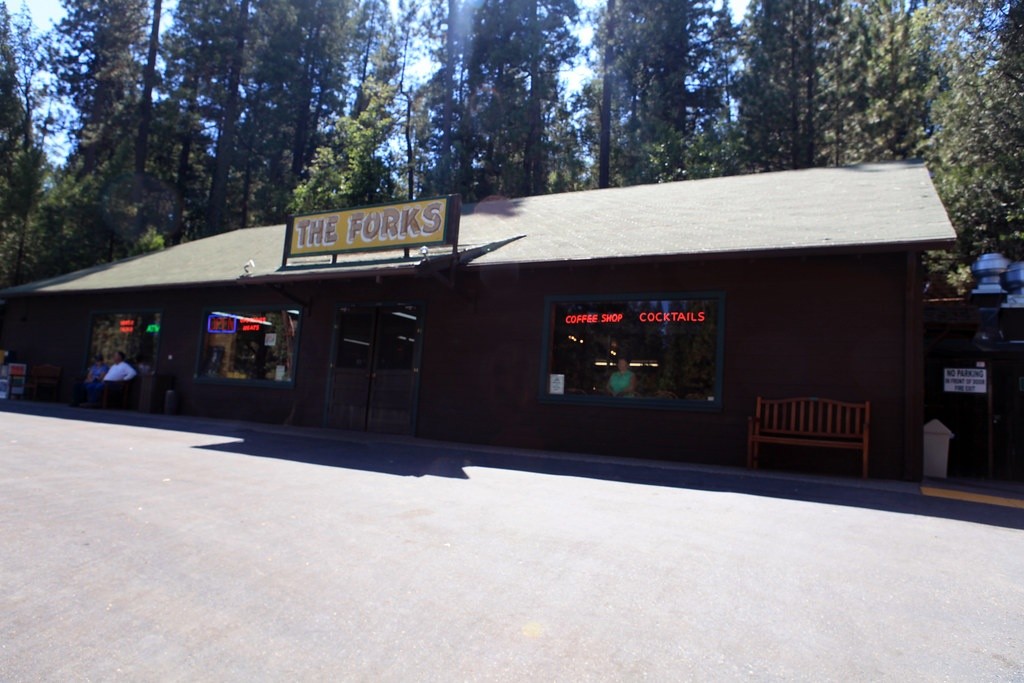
[746,395,871,479]
[78,368,132,411]
[28,366,62,403]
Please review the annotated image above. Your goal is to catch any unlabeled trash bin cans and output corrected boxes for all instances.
[142,364,178,414]
[923,418,954,479]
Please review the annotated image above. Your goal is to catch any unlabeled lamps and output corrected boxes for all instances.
[420,246,430,262]
[240,258,254,277]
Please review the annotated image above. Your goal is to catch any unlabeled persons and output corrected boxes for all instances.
[606,356,636,398]
[80,351,137,407]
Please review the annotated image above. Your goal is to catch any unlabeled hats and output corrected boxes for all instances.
[94,352,103,361]
